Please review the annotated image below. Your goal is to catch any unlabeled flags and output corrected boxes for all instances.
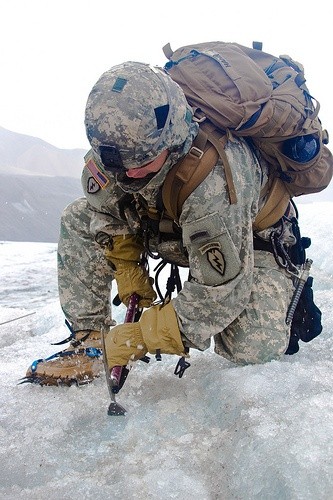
[85,157,110,190]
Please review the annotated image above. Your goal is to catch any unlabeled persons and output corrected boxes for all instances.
[18,61,323,388]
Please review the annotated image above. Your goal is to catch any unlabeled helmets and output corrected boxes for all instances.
[85,60,189,173]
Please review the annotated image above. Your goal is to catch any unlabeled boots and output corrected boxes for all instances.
[26,327,112,387]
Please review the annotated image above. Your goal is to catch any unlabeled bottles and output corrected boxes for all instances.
[289,135,317,162]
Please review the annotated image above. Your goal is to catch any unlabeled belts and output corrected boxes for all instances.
[253,200,291,249]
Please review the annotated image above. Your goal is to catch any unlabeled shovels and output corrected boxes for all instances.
[97,292,141,418]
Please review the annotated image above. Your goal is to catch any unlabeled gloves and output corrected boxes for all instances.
[105,297,190,369]
[102,235,157,308]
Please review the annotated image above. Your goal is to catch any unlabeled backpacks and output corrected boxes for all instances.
[162,41,333,232]
